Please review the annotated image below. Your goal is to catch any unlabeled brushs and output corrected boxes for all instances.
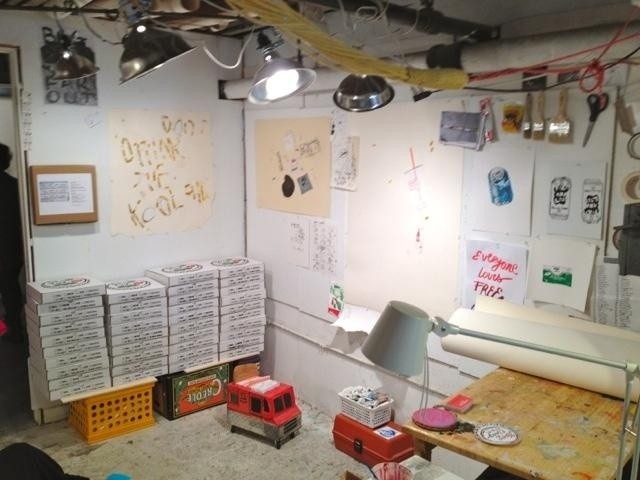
[523,87,573,143]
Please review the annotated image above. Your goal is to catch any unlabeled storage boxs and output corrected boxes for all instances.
[231,355,262,383]
[24,256,268,402]
[331,414,413,468]
[153,364,230,420]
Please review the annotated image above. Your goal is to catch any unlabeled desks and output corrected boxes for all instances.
[399,367,639,480]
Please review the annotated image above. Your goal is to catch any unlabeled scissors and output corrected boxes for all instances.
[583,93,610,147]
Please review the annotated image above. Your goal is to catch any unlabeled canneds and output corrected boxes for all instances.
[488,167,513,206]
[548,175,571,220]
[581,176,603,223]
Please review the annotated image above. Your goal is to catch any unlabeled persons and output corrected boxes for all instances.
[0,143,25,343]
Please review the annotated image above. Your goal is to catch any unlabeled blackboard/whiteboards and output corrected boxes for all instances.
[243,84,622,398]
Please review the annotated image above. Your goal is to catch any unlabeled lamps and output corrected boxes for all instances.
[50,2,396,115]
[359,299,639,479]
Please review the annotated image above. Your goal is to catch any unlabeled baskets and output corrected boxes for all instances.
[338,385,394,430]
